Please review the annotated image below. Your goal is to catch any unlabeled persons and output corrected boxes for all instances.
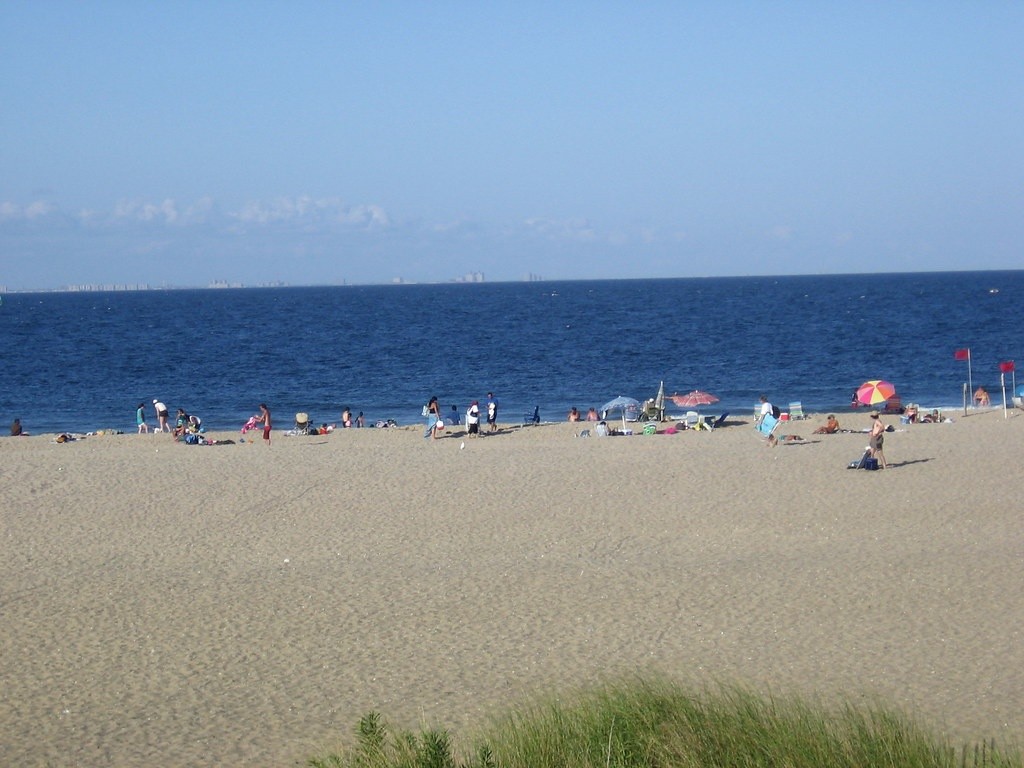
[756,396,801,447]
[868,410,886,469]
[296,412,336,434]
[11,419,23,436]
[904,406,944,424]
[568,407,600,421]
[137,399,199,437]
[343,408,364,429]
[813,414,842,434]
[600,421,613,434]
[974,387,989,406]
[852,388,860,406]
[425,392,499,439]
[254,404,271,445]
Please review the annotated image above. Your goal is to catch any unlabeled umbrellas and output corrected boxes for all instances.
[598,396,640,431]
[675,389,720,413]
[655,381,665,421]
[857,380,896,405]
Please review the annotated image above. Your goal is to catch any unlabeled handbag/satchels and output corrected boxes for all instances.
[468,415,479,424]
[422,405,430,418]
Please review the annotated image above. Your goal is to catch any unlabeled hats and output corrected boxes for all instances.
[296,413,308,423]
[153,400,157,404]
[871,410,880,416]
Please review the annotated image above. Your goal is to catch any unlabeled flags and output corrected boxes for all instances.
[955,348,968,360]
[1000,361,1015,372]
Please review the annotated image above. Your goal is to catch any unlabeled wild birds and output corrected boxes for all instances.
[461,441,465,450]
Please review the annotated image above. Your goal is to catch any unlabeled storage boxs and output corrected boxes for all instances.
[781,413,789,420]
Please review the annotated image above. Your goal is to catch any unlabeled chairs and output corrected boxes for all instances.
[713,412,729,428]
[750,413,782,443]
[789,401,809,421]
[594,423,616,437]
[647,407,659,421]
[684,410,701,430]
[753,403,762,421]
[904,403,920,423]
[704,415,715,426]
[295,412,314,437]
[625,409,639,422]
[886,396,901,415]
[928,408,942,423]
